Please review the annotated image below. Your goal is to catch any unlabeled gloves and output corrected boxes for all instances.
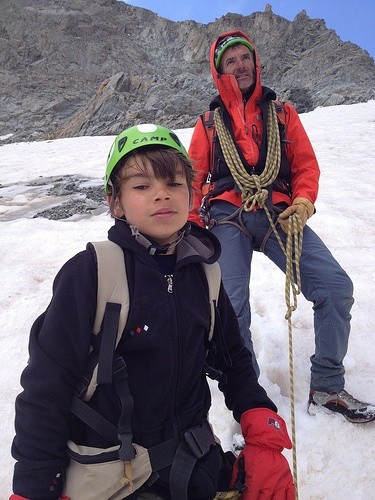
[277,198,314,233]
[230,408,297,500]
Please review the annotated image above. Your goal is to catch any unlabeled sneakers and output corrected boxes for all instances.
[309,388,375,423]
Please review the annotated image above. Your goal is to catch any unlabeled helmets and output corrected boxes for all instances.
[105,124,193,191]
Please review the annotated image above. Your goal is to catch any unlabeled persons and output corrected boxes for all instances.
[188,30,375,423]
[9,123,296,500]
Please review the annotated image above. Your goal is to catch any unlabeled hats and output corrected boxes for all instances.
[216,36,255,68]
